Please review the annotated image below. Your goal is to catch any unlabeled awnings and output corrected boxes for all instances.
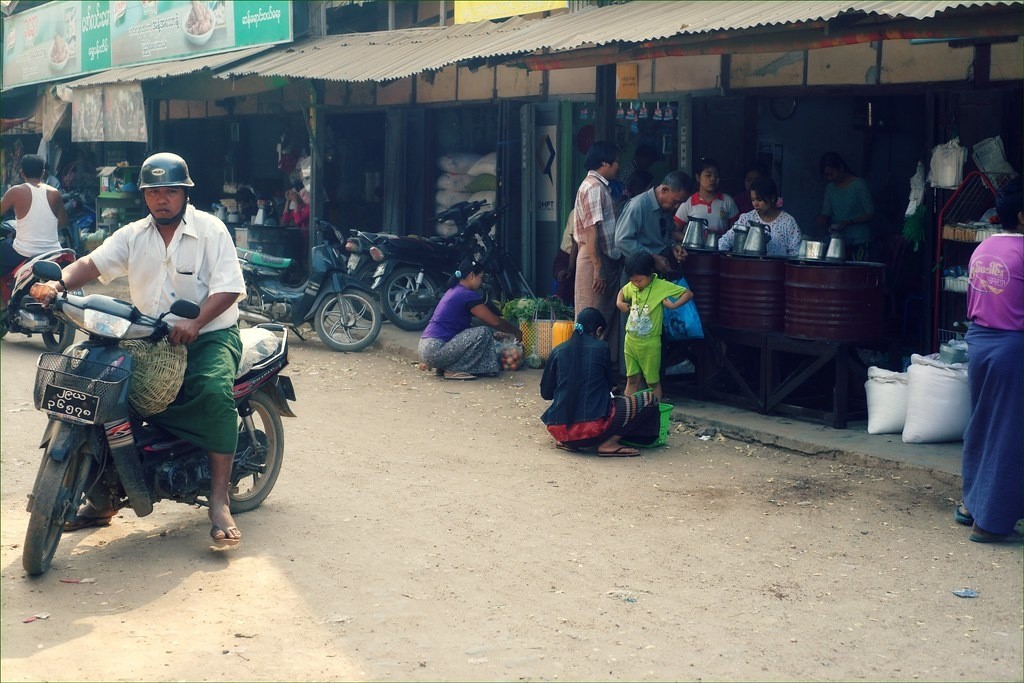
[59,0,1023,87]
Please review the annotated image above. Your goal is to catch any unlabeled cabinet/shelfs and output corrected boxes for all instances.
[930,168,1018,353]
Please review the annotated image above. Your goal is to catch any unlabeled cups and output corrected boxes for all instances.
[228,214,239,222]
[797,239,826,260]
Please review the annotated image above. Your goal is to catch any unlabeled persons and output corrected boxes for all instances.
[819,151,875,260]
[540,307,661,457]
[718,179,801,257]
[556,141,622,373]
[0,154,68,276]
[614,170,692,383]
[728,162,772,229]
[418,260,522,380]
[616,253,693,402]
[279,184,317,239]
[42,163,62,191]
[672,158,741,242]
[29,152,248,545]
[952,178,1024,543]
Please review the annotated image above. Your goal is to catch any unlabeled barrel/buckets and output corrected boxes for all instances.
[716,252,785,333]
[783,260,887,342]
[678,252,717,326]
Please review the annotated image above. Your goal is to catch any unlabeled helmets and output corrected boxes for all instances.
[138,153,195,191]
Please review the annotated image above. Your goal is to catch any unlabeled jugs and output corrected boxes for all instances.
[825,234,847,262]
[212,199,274,225]
[679,216,772,256]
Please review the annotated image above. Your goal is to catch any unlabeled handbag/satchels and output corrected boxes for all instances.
[498,337,524,371]
[660,261,705,344]
[519,295,576,370]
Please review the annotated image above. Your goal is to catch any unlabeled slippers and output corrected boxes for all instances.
[596,446,641,458]
[954,504,974,527]
[444,372,477,379]
[62,516,113,532]
[0,309,10,322]
[555,441,581,452]
[210,525,242,551]
[969,529,1024,544]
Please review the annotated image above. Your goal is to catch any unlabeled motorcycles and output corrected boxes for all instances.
[20,260,298,576]
[1,217,85,356]
[341,198,521,330]
[234,216,383,352]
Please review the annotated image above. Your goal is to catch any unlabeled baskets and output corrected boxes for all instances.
[617,387,674,447]
[34,352,131,427]
[118,335,188,419]
